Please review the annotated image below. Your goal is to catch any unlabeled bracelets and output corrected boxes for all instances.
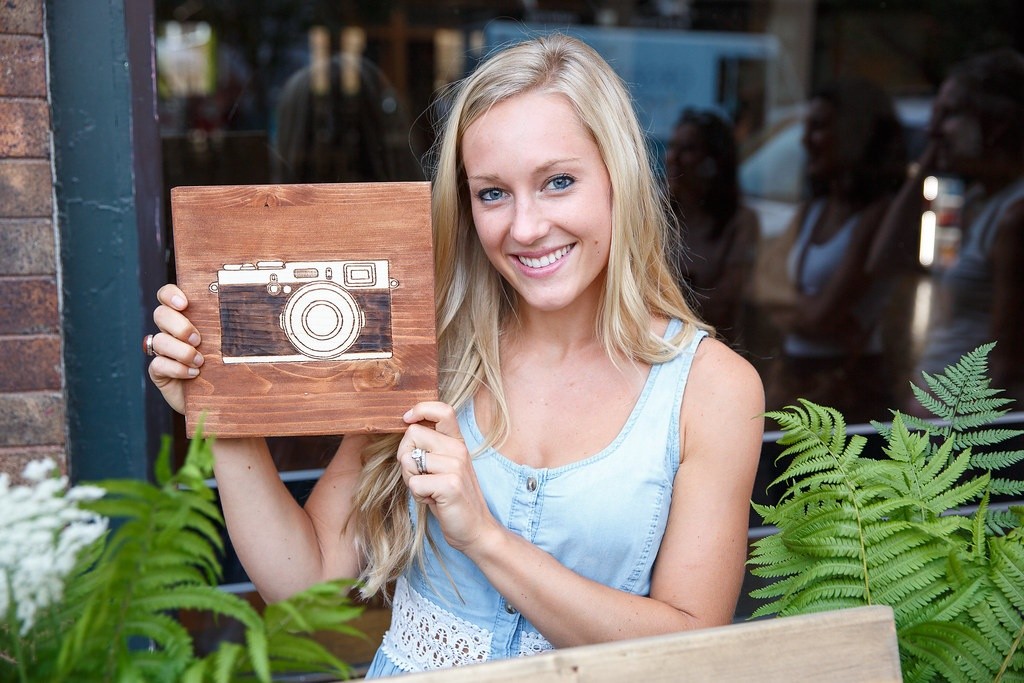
[909,160,929,183]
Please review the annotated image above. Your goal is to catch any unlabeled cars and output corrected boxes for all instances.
[728,93,950,272]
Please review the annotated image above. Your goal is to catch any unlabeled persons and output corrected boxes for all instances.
[864,53,1023,413]
[270,54,420,183]
[144,33,766,678]
[745,73,908,426]
[656,103,759,354]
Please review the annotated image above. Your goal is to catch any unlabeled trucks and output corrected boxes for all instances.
[482,23,804,184]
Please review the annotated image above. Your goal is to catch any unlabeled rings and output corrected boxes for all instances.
[411,449,429,475]
[144,335,160,356]
